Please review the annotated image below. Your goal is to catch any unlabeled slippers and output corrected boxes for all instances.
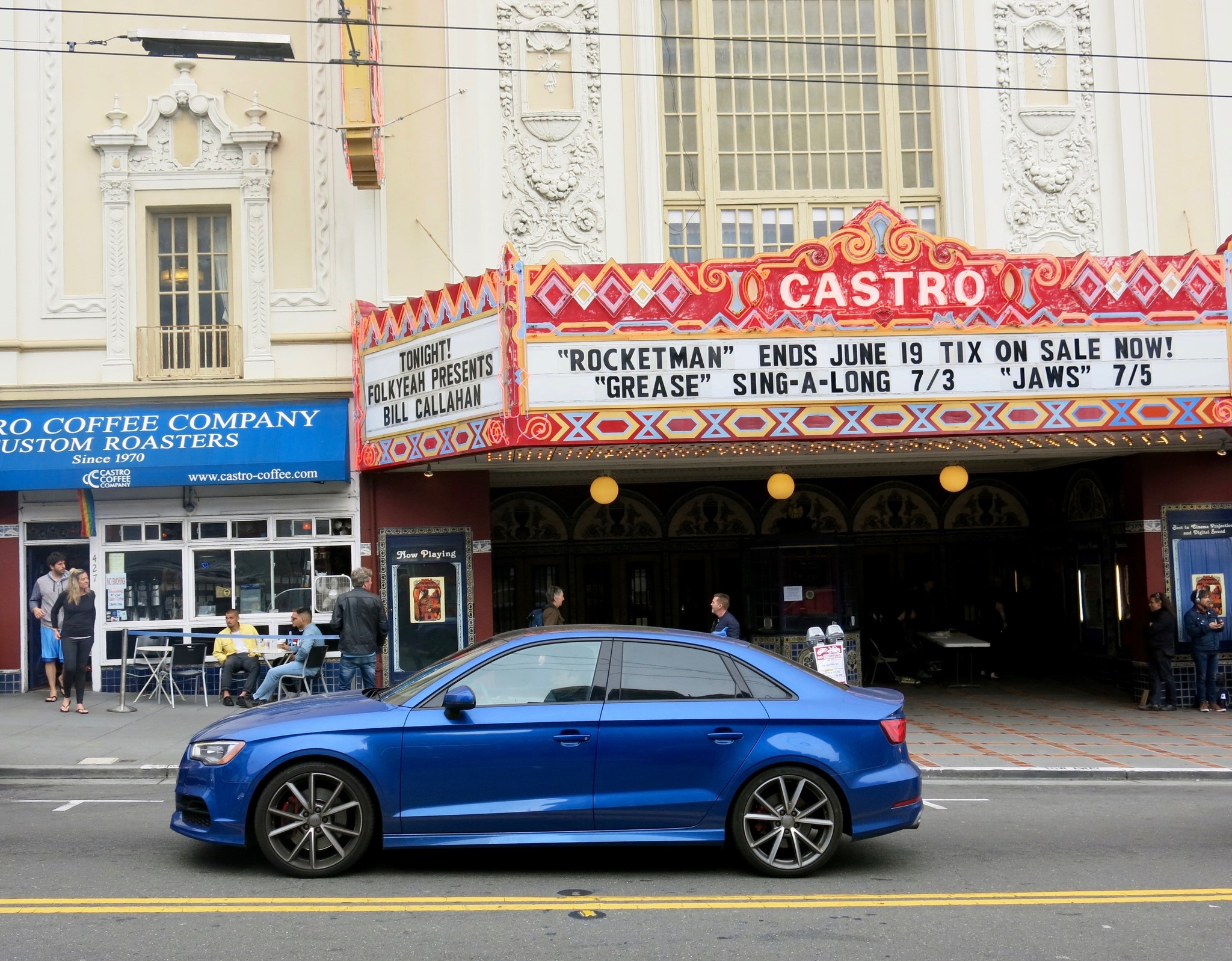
[60,700,71,712]
[76,709,89,714]
[45,696,58,702]
[58,680,66,696]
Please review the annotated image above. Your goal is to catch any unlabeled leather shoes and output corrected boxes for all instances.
[236,696,245,706]
[1138,704,1158,711]
[1160,705,1176,711]
[223,696,234,706]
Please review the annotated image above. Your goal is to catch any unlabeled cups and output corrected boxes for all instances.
[277,641,284,652]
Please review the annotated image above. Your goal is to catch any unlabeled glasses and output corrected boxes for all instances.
[291,616,296,620]
[553,587,558,596]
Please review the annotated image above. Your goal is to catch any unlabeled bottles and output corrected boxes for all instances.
[257,639,261,651]
[172,594,183,620]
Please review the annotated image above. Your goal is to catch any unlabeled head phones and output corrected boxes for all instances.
[1194,589,1201,604]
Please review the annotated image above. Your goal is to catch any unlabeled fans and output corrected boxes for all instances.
[314,575,352,613]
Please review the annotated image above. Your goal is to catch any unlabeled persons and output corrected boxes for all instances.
[1137,593,1178,711]
[710,593,739,639]
[51,567,96,713]
[29,553,70,703]
[213,609,264,706]
[244,607,325,711]
[330,567,389,691]
[1183,591,1227,712]
[894,580,943,684]
[537,587,565,691]
[980,600,1016,679]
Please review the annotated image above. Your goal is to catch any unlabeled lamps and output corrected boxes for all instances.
[424,463,433,477]
[1217,438,1226,456]
[160,265,204,287]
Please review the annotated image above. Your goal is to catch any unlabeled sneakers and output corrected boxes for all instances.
[1209,703,1227,712]
[244,699,266,708]
[1199,700,1209,712]
[243,696,259,706]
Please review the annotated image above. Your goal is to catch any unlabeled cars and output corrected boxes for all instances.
[170,623,923,879]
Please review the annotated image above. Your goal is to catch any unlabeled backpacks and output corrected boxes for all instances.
[526,606,555,627]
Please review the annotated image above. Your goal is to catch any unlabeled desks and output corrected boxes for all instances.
[918,630,991,687]
[133,646,192,704]
[250,648,295,698]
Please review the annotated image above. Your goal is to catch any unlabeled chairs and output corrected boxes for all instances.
[278,645,329,702]
[864,638,902,685]
[218,666,260,702]
[127,635,169,699]
[158,643,209,709]
